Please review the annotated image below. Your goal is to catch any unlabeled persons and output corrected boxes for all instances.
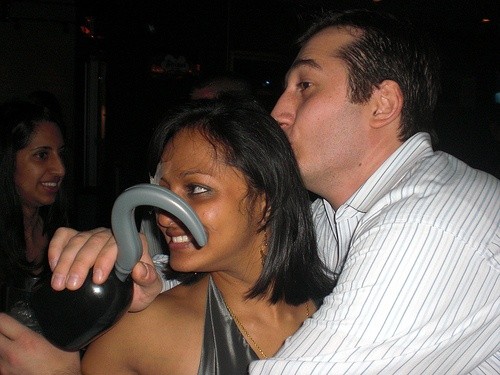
[80,94,336,375]
[0,24,499,375]
[0,111,75,333]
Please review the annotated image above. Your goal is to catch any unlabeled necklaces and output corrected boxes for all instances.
[216,278,312,361]
